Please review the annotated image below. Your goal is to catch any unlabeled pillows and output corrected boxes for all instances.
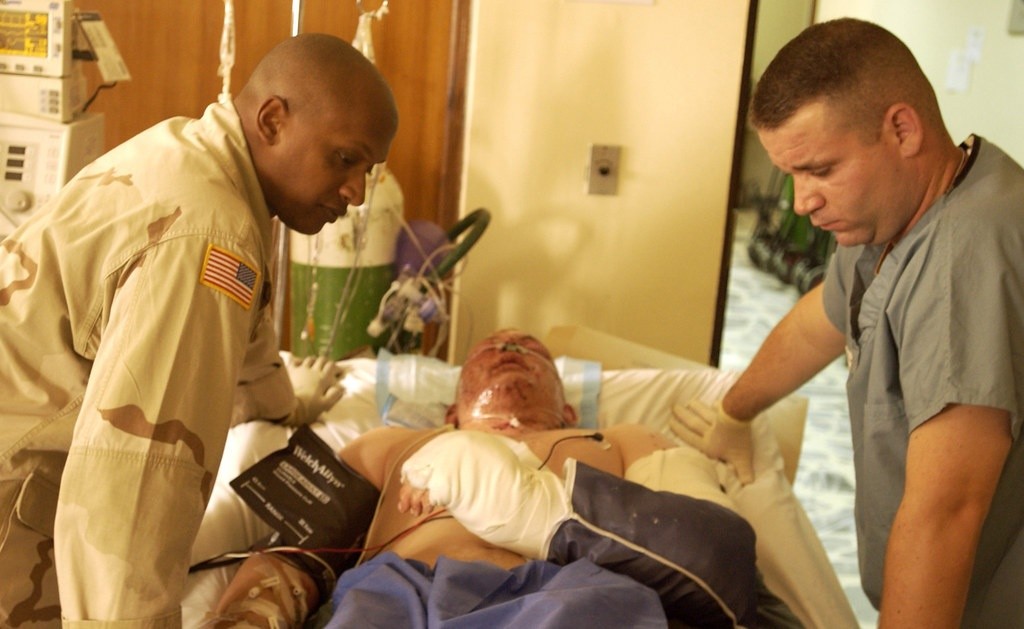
[184,357,811,487]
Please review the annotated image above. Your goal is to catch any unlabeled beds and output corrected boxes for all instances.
[177,478,866,629]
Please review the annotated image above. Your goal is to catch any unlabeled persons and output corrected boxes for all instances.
[201,328,804,628]
[0,32,399,629]
[667,24,1024,629]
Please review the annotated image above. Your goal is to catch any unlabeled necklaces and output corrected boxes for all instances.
[946,146,966,194]
